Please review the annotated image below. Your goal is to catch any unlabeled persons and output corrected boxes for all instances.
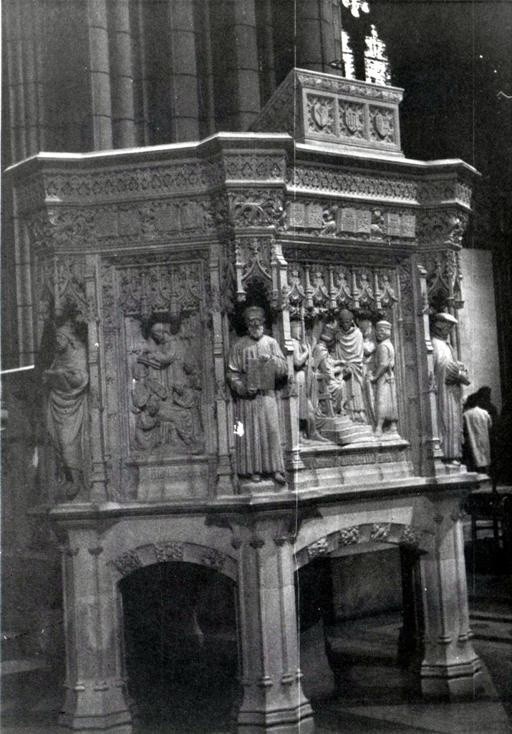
[43,315,91,498]
[134,322,197,446]
[465,387,512,484]
[226,305,287,486]
[291,309,399,443]
[432,312,471,466]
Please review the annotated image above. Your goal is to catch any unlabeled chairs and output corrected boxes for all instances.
[468,456,510,553]
